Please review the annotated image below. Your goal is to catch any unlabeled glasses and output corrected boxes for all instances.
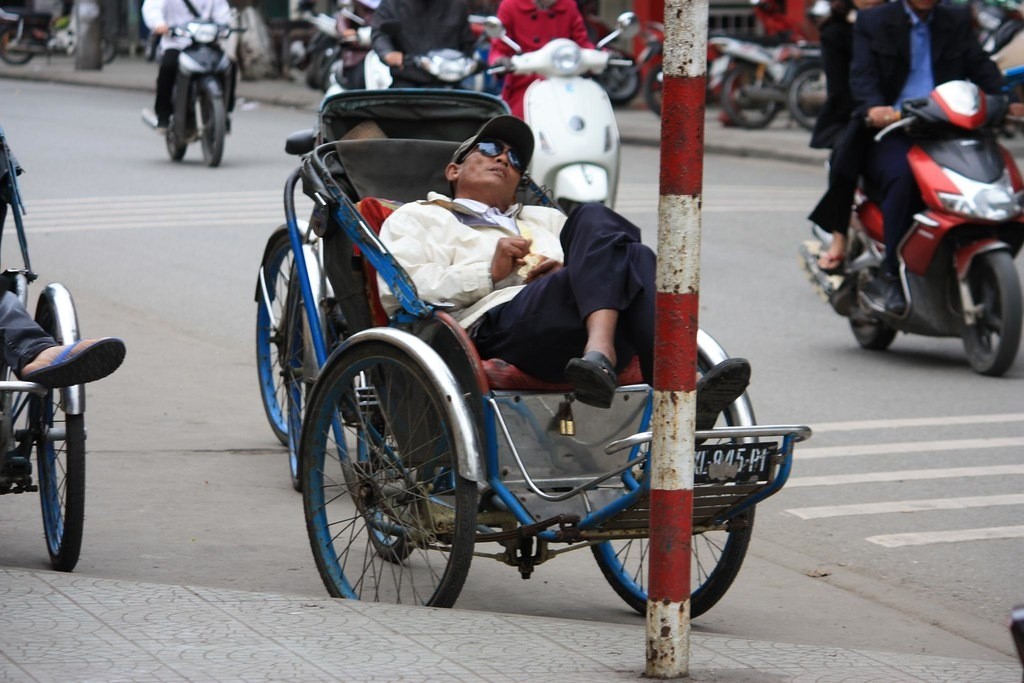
[460,139,523,173]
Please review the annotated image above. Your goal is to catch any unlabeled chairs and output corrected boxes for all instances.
[357,195,656,393]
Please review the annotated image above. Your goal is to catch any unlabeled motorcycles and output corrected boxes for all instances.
[293,9,490,92]
[584,16,637,103]
[0,4,118,68]
[140,18,247,168]
[637,22,827,130]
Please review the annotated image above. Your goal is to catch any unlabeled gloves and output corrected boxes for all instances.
[402,53,428,71]
[490,57,515,80]
[603,55,627,73]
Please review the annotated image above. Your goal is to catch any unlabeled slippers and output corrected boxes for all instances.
[695,358,750,443]
[821,253,844,271]
[23,337,126,389]
[565,352,617,408]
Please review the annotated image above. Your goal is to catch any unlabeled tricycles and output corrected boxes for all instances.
[252,87,813,618]
[0,124,87,572]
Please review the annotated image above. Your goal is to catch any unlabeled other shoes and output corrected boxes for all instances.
[158,112,169,128]
[224,116,231,132]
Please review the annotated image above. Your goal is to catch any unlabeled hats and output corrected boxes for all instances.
[450,115,535,174]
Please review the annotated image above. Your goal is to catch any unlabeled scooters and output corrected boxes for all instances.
[481,11,641,211]
[800,79,1024,377]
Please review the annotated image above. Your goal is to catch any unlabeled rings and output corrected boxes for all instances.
[883,115,891,120]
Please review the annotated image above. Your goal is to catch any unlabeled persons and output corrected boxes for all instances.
[375,115,751,446]
[371,0,485,92]
[0,284,127,390]
[489,0,623,119]
[140,0,240,133]
[807,0,1024,314]
